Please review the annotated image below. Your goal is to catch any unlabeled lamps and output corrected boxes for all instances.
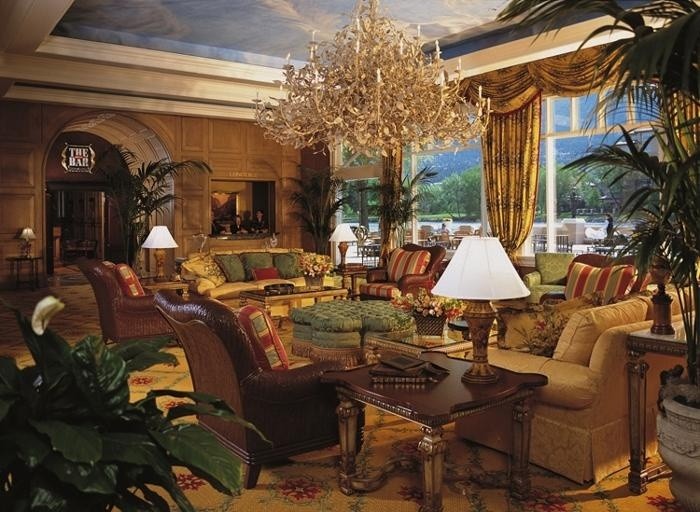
[19,226,38,257]
[429,236,530,384]
[328,223,357,269]
[141,225,179,280]
[252,1,491,156]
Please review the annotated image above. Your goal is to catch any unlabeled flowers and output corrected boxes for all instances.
[300,251,333,278]
[391,287,466,319]
[0,297,274,512]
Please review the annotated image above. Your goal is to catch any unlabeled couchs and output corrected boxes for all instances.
[154,289,363,489]
[291,300,419,367]
[78,257,183,347]
[180,247,335,298]
[350,242,446,301]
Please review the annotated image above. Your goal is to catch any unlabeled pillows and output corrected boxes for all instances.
[453,250,698,497]
[387,247,431,282]
[240,251,273,280]
[252,268,279,281]
[231,305,289,371]
[182,256,226,287]
[214,254,247,281]
[114,263,144,297]
[271,253,298,278]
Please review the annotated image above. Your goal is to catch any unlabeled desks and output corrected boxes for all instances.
[8,255,44,290]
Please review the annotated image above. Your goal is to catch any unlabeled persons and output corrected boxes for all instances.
[436,223,452,247]
[601,213,614,237]
[211,209,271,236]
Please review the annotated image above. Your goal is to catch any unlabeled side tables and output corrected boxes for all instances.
[332,267,367,293]
[138,277,189,299]
[318,349,549,511]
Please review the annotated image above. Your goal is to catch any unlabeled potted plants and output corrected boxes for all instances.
[558,117,698,507]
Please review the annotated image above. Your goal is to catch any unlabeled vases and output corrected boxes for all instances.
[413,310,447,337]
[302,271,325,290]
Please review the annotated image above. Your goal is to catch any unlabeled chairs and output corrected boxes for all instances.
[349,223,558,255]
[66,239,98,258]
